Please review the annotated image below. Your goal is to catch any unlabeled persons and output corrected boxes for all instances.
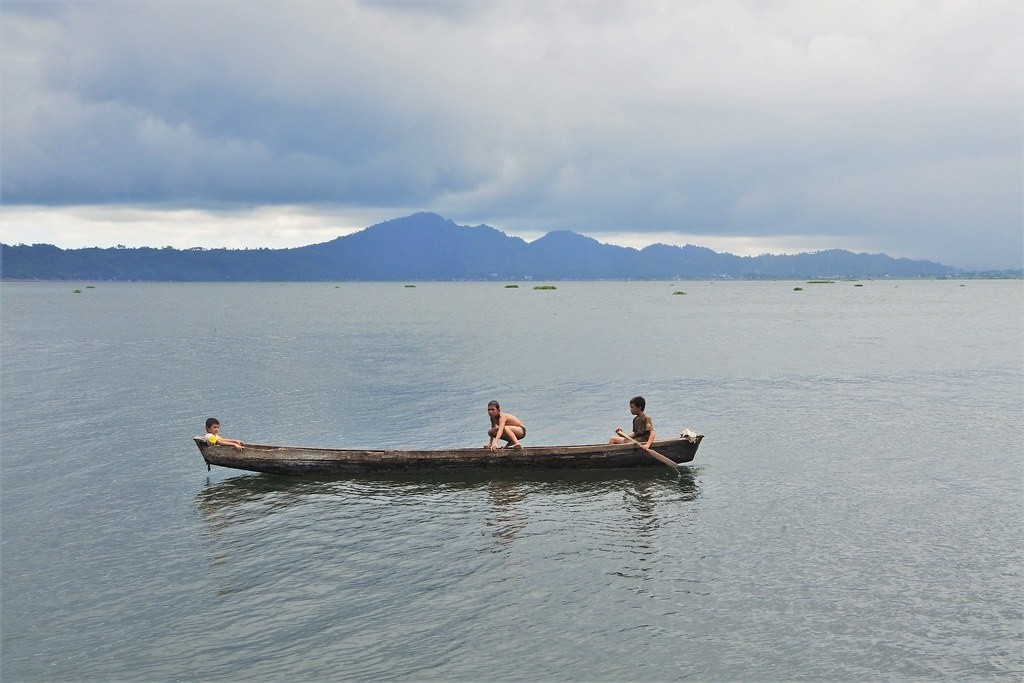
[608,395,655,449]
[204,418,244,452]
[482,400,526,452]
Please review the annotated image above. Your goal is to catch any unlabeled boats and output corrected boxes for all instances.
[191,434,706,473]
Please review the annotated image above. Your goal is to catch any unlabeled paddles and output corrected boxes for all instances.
[617,431,679,469]
